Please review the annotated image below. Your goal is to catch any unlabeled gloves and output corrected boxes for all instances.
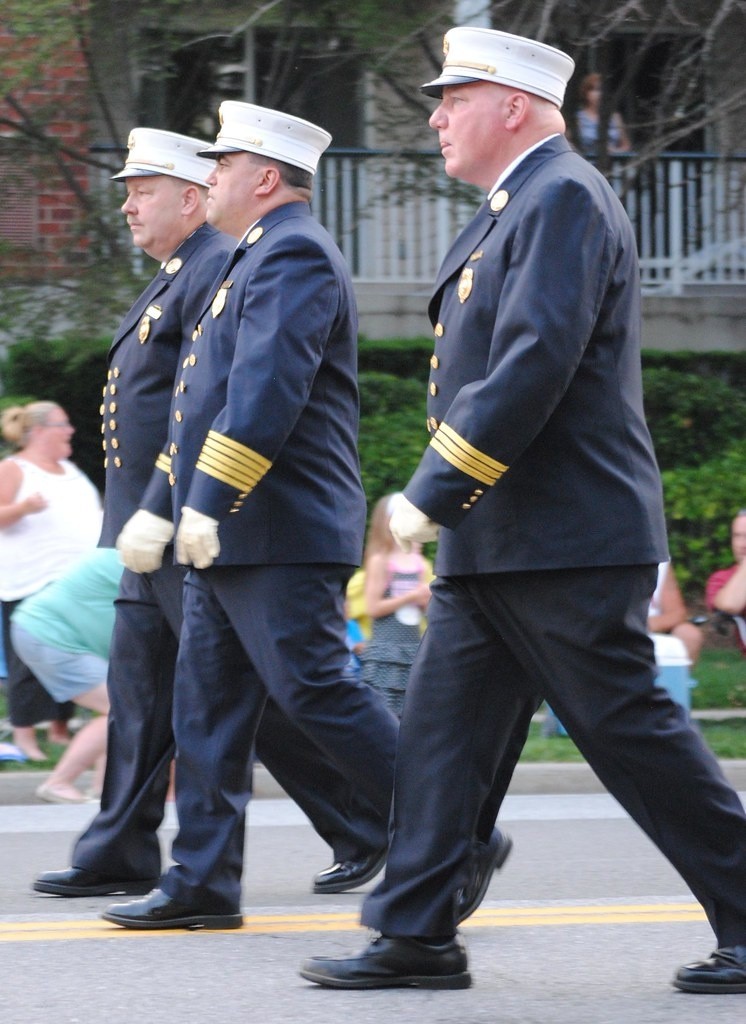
[115,509,175,575]
[388,496,442,554]
[176,506,220,568]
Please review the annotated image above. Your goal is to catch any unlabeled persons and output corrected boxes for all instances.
[345,489,438,724]
[32,125,392,898]
[568,70,632,156]
[101,96,514,931]
[0,396,126,807]
[294,23,746,996]
[648,511,746,715]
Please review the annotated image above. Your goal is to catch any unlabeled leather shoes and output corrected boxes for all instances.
[34,866,160,896]
[300,930,471,990]
[313,848,390,894]
[102,888,243,930]
[674,941,746,993]
[455,824,513,925]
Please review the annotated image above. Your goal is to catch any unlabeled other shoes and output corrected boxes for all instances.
[36,785,91,802]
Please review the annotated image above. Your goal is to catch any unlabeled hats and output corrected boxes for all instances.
[196,100,333,176]
[420,27,577,109]
[110,128,220,188]
